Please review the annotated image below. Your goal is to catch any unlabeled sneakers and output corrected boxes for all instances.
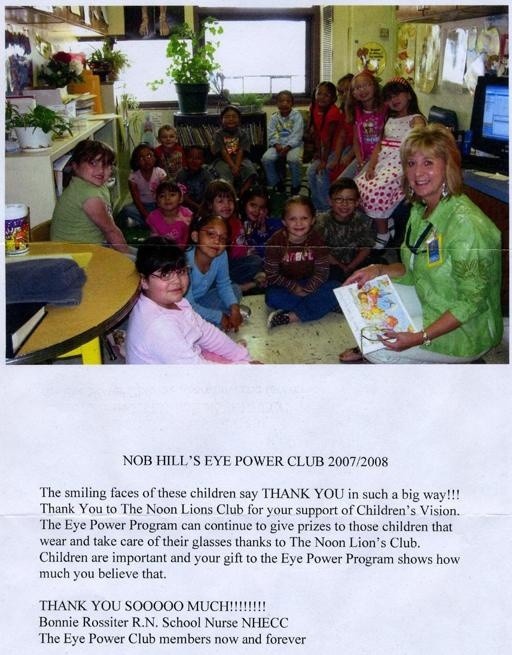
[252,272,267,286]
[239,303,251,320]
[266,307,291,330]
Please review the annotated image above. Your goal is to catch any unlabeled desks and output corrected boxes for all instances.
[5,241,142,364]
[461,169,509,314]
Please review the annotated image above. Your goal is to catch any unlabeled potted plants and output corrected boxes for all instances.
[5,99,73,149]
[229,93,271,112]
[166,16,224,114]
[86,40,133,84]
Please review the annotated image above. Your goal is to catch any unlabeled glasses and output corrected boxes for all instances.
[331,196,356,204]
[148,269,191,282]
[201,231,232,241]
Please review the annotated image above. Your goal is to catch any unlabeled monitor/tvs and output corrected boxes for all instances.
[467,75,508,160]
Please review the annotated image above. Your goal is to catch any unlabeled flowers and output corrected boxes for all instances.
[36,58,86,89]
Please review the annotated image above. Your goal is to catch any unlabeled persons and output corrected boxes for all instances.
[48,72,504,365]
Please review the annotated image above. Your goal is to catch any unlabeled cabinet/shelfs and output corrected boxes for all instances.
[27,6,108,35]
[49,117,120,210]
[173,112,268,154]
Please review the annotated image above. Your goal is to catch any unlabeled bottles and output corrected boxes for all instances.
[5,203,30,257]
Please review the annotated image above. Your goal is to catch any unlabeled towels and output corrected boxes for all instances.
[6,259,86,307]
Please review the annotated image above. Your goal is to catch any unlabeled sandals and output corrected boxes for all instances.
[373,232,391,256]
[388,219,400,242]
[338,346,366,362]
